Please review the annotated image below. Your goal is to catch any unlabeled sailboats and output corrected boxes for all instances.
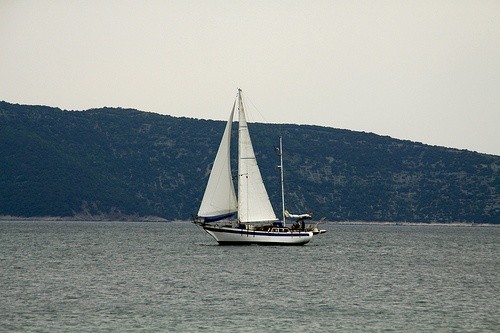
[191,88,326,245]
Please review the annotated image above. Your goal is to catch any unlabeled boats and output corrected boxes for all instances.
[281,210,327,235]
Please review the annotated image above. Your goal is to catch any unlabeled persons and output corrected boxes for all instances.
[301,220,305,231]
